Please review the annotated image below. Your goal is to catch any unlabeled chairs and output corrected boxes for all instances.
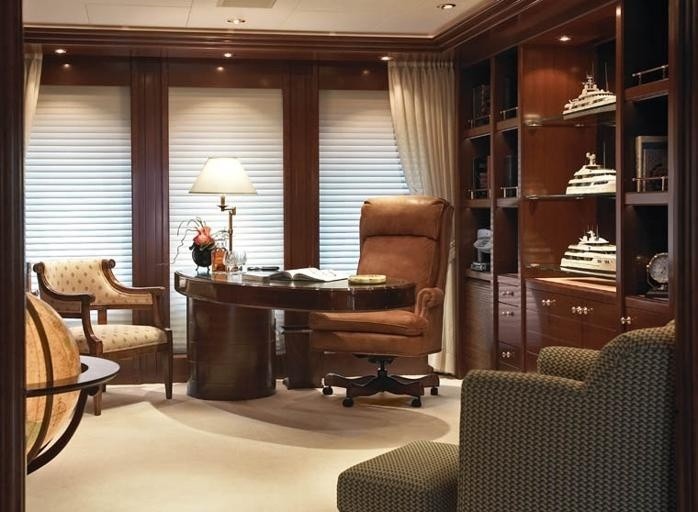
[309,194,454,408]
[32,255,173,415]
[336,317,676,512]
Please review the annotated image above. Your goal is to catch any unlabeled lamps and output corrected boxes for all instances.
[188,155,259,251]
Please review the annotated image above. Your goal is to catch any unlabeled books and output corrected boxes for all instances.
[469,69,517,199]
[634,134,667,192]
[241,267,347,282]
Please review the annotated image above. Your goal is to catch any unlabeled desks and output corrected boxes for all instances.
[173,268,419,402]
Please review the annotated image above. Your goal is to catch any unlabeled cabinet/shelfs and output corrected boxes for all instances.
[453,0,698,381]
